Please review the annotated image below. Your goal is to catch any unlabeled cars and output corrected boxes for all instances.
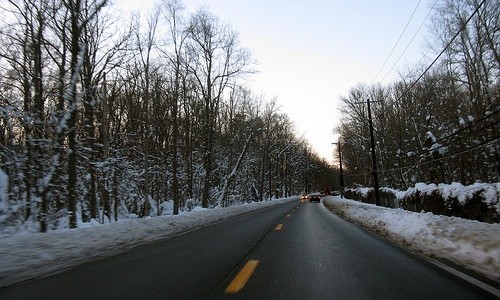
[309,195,321,203]
[320,189,341,196]
[301,195,308,199]
